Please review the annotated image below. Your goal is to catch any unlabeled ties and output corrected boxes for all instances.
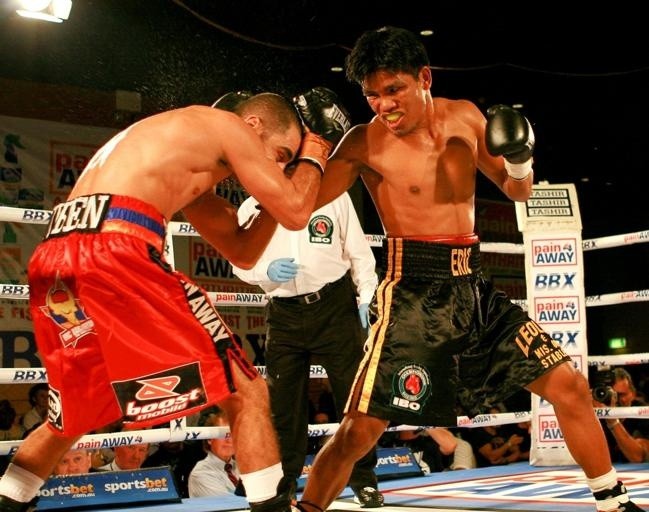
[225,465,238,487]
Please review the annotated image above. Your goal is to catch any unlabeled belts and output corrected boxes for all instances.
[273,274,349,304]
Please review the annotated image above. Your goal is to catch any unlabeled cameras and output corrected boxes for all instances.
[589,371,622,407]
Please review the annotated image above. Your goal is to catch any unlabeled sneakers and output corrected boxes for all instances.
[354,487,384,508]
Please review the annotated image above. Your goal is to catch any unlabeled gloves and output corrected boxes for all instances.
[210,91,254,110]
[293,87,352,173]
[359,303,370,329]
[267,258,298,282]
[485,104,535,179]
